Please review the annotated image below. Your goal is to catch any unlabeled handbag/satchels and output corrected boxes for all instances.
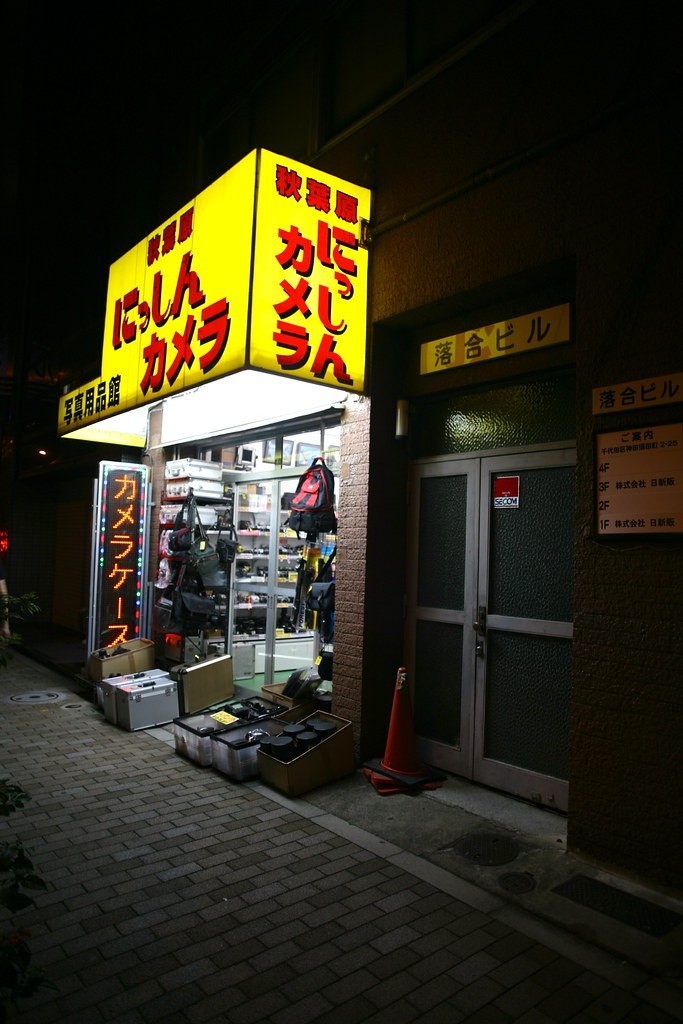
[306,545,336,612]
[281,492,294,509]
[317,631,333,681]
[165,489,239,624]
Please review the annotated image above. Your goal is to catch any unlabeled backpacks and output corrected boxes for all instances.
[290,457,337,540]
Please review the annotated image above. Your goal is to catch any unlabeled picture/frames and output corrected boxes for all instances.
[296,442,323,467]
[263,438,294,466]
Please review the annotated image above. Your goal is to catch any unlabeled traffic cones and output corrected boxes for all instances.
[361,667,447,794]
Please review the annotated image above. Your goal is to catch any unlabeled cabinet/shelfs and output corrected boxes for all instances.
[205,481,336,640]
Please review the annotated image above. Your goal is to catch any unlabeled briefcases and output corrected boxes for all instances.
[117,677,179,731]
[170,651,234,714]
[101,668,170,726]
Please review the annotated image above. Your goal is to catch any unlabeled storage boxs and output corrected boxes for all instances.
[210,717,295,782]
[88,637,156,683]
[261,682,301,708]
[256,709,352,797]
[172,695,289,770]
